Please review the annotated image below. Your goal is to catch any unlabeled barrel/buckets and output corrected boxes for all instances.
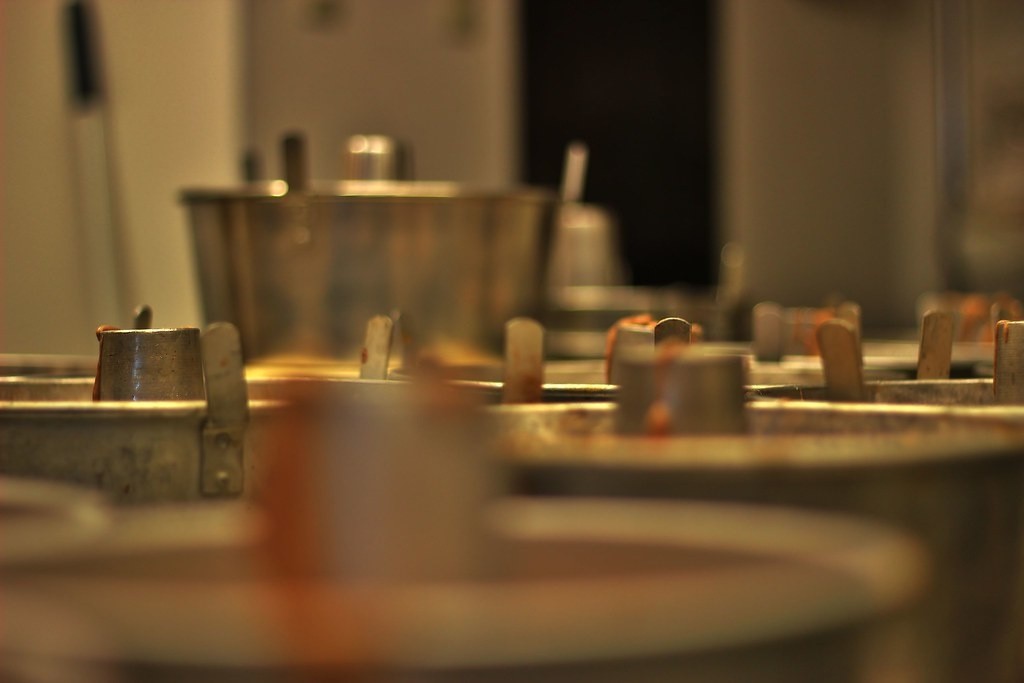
[0,130,1024,683]
